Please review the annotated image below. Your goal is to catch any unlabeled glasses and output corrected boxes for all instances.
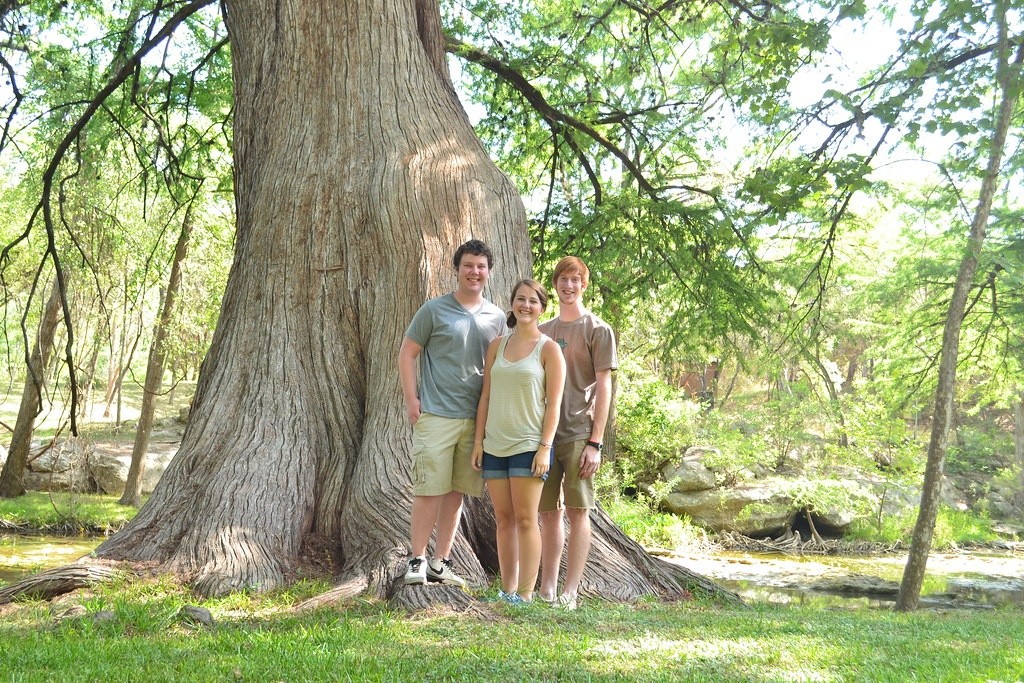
[555,275,583,283]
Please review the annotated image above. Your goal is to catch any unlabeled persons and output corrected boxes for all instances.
[398,240,509,584]
[472,279,566,607]
[538,256,617,607]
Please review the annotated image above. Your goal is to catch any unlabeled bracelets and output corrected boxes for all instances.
[540,441,551,448]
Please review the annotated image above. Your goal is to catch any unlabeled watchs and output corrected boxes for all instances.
[587,440,603,450]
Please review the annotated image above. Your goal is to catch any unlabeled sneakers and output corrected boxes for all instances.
[541,593,577,611]
[425,557,465,588]
[480,590,531,605]
[404,556,427,584]
